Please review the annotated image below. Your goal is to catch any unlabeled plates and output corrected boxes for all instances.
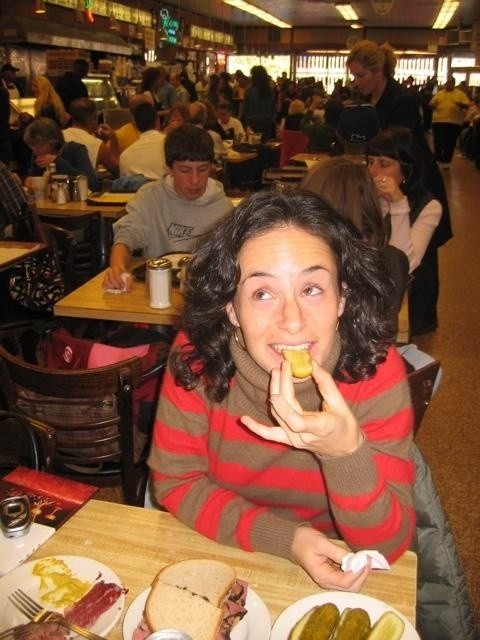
[271,590,421,640]
[0,556,124,640]
[123,584,272,640]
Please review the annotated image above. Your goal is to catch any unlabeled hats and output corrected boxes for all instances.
[2,64,19,72]
[338,100,379,142]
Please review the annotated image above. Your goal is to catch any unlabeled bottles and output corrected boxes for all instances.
[72,175,87,200]
[57,183,66,204]
[48,162,56,198]
[145,257,171,310]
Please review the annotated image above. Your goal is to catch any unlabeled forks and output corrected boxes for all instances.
[8,588,111,640]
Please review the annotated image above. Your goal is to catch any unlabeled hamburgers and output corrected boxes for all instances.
[132,560,247,640]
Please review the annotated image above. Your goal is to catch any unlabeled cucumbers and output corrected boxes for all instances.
[286,601,405,640]
[281,348,313,378]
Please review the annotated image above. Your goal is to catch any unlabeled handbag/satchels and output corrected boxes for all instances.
[9,205,65,312]
[15,328,168,463]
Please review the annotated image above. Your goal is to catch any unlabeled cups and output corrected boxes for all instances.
[50,174,70,203]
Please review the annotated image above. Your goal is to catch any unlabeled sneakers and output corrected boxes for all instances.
[411,317,437,334]
[437,152,466,170]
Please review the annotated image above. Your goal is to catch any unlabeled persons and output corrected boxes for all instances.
[148,185,423,595]
[0,154,69,324]
[363,126,443,275]
[102,122,236,293]
[298,155,411,344]
[1,39,479,198]
[346,40,453,337]
